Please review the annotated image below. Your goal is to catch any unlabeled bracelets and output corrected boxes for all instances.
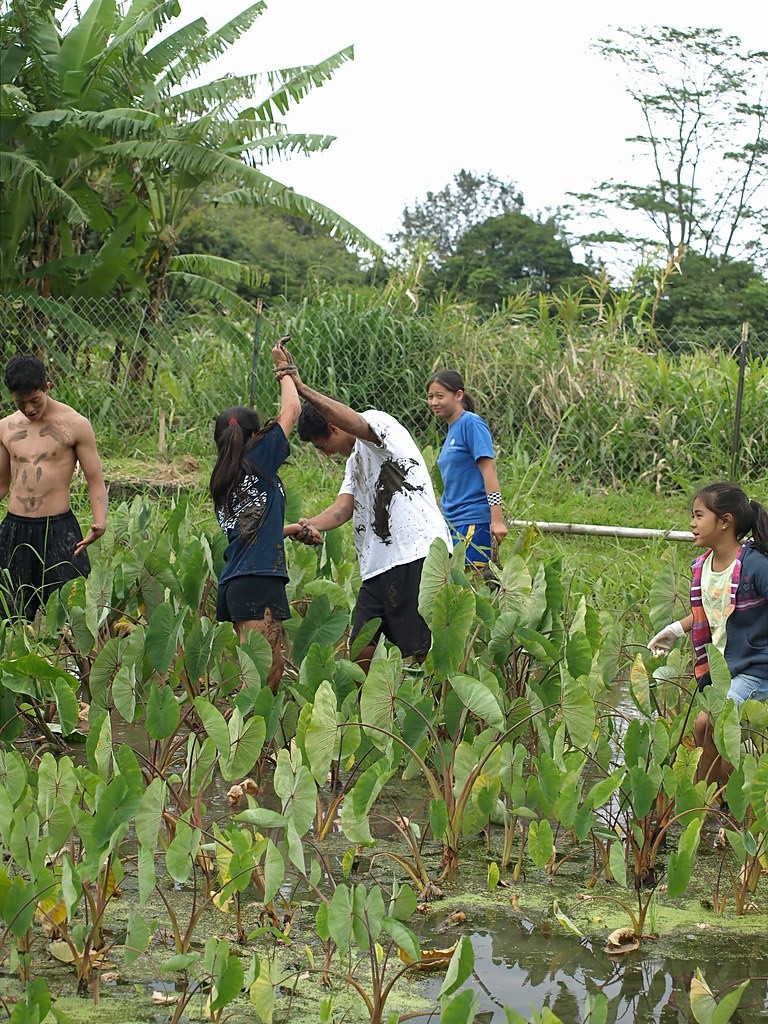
[487,492,502,506]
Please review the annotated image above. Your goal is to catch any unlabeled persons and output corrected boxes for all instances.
[272,346,454,739]
[648,483,767,848]
[1,355,108,733]
[426,370,508,579]
[209,334,323,724]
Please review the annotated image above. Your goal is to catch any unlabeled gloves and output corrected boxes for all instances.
[647,621,687,659]
